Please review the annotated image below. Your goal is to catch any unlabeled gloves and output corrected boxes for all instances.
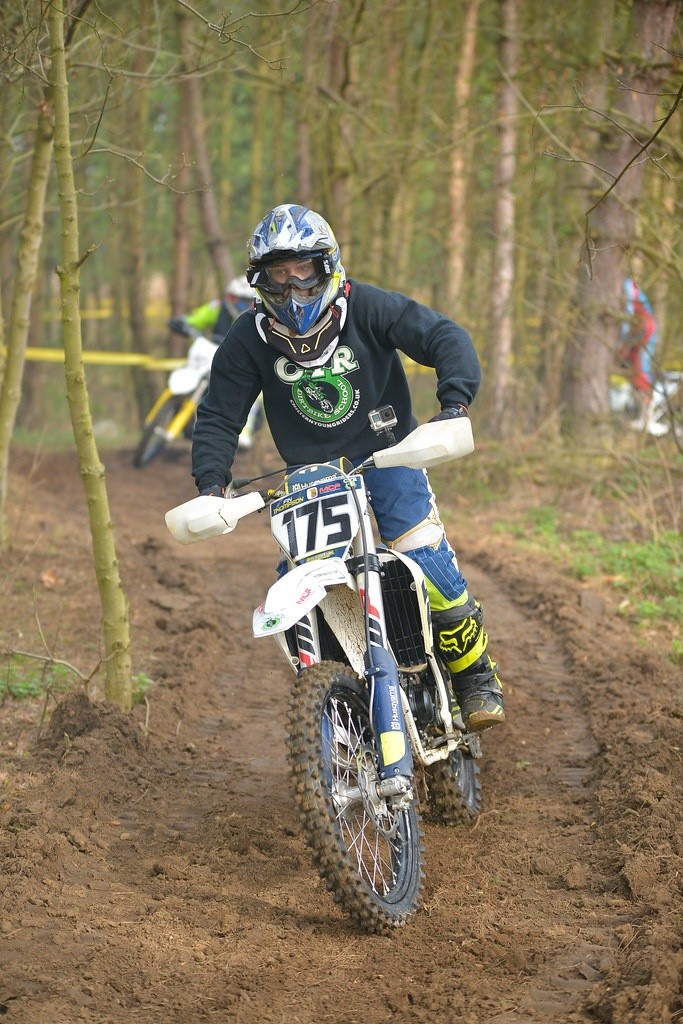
[197,485,225,498]
[430,404,466,423]
[168,316,194,338]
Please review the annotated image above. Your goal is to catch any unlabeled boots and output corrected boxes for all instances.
[430,598,505,726]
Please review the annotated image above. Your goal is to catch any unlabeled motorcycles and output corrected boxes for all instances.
[613,371,680,441]
[133,331,266,471]
[165,414,488,940]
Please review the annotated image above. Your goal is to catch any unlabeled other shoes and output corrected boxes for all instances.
[238,433,258,448]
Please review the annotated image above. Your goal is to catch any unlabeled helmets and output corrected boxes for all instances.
[244,204,344,335]
[224,274,254,321]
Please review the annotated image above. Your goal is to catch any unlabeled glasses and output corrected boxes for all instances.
[229,297,254,311]
[246,248,341,295]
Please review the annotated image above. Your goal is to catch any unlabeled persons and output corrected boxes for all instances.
[622,257,659,431]
[169,275,263,449]
[192,203,507,734]
